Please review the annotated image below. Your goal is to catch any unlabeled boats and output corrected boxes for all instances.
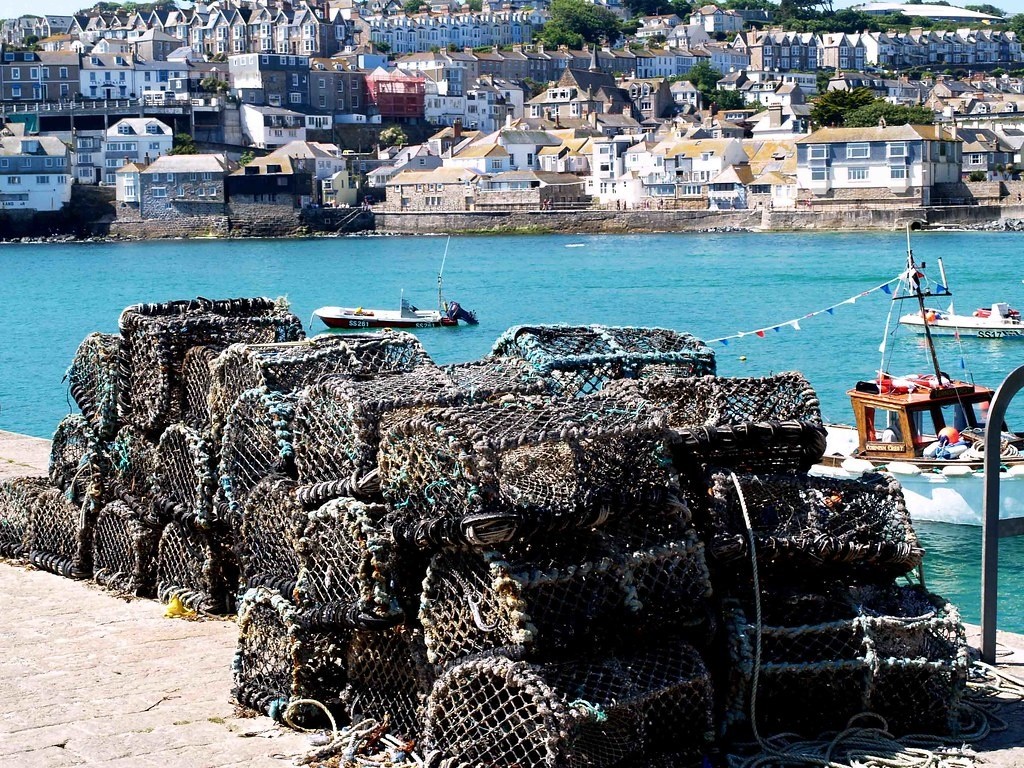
[898,302,1024,339]
[308,235,480,331]
[806,221,1024,528]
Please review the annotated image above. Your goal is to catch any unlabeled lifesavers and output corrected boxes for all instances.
[865,371,954,398]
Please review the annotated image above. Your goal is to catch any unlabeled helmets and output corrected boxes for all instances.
[938,427,959,445]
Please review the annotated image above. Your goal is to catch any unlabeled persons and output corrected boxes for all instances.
[541,199,552,210]
[1018,194,1021,203]
[923,310,942,321]
[617,197,813,212]
[1004,309,1017,324]
[882,422,902,442]
